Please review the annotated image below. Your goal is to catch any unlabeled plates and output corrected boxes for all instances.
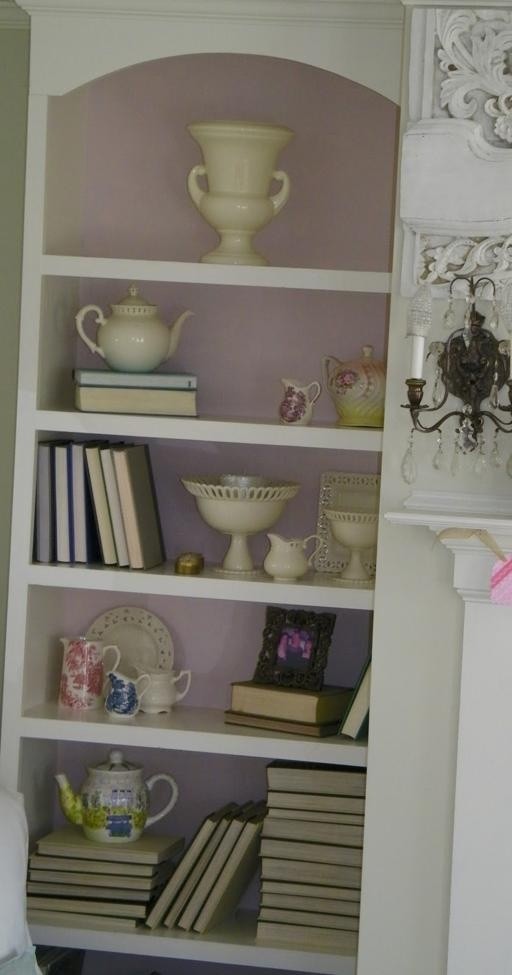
[85,606,175,700]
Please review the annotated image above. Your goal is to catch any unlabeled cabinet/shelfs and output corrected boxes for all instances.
[0,0,512,975]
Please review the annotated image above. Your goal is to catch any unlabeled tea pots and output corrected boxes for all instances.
[54,750,179,845]
[76,283,193,373]
[322,345,385,428]
[278,376,322,426]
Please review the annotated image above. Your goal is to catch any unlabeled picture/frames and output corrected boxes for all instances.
[317,472,381,573]
[253,607,336,690]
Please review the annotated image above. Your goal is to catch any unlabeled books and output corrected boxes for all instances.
[27,828,185,932]
[144,799,269,933]
[32,439,167,572]
[337,657,372,740]
[254,759,366,958]
[72,368,198,418]
[224,682,355,737]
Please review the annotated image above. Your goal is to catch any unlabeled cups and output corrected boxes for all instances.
[57,636,121,713]
[134,665,192,715]
[264,533,323,581]
[104,670,151,719]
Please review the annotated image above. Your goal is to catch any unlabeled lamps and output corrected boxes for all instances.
[402,271,512,482]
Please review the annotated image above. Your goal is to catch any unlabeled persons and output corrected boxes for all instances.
[278,630,313,668]
